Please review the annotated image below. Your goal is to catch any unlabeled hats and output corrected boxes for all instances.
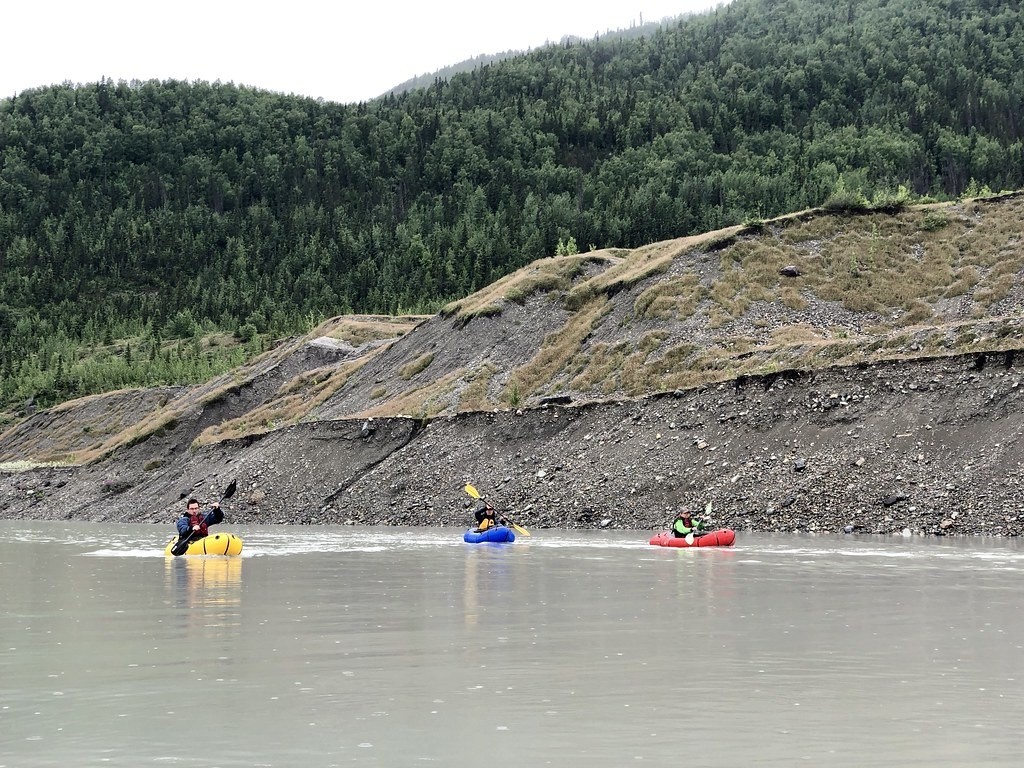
[680,508,690,513]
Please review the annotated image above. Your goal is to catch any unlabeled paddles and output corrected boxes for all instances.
[465,483,530,536]
[171,479,236,556]
[685,502,711,545]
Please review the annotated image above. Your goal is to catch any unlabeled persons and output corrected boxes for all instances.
[177,498,224,542]
[673,507,706,538]
[475,503,508,529]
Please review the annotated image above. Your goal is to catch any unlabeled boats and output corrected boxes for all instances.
[463,527,515,543]
[648,528,735,548]
[165,533,244,556]
